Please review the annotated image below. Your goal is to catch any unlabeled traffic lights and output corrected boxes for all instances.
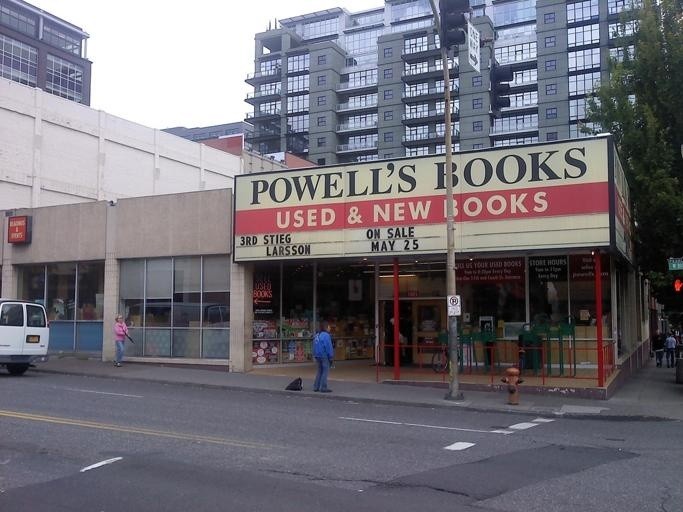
[671,276,683,301]
[440,0,469,50]
[491,64,513,118]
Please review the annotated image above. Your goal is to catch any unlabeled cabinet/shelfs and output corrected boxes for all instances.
[251,316,376,366]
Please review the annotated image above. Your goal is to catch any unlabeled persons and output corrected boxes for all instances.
[662,331,677,368]
[652,334,663,368]
[112,314,128,367]
[659,327,681,352]
[310,321,335,393]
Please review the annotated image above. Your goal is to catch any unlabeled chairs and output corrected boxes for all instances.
[436,313,577,378]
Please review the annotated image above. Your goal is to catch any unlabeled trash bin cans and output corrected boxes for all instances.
[676,359,683,383]
[520,335,544,370]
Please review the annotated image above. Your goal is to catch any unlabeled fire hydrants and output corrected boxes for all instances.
[500,367,525,405]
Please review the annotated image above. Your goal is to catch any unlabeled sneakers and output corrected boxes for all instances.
[113,359,121,367]
[314,389,332,392]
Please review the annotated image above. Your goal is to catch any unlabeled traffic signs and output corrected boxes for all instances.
[667,257,683,270]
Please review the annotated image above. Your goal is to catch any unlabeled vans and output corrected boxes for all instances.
[0,298,50,377]
